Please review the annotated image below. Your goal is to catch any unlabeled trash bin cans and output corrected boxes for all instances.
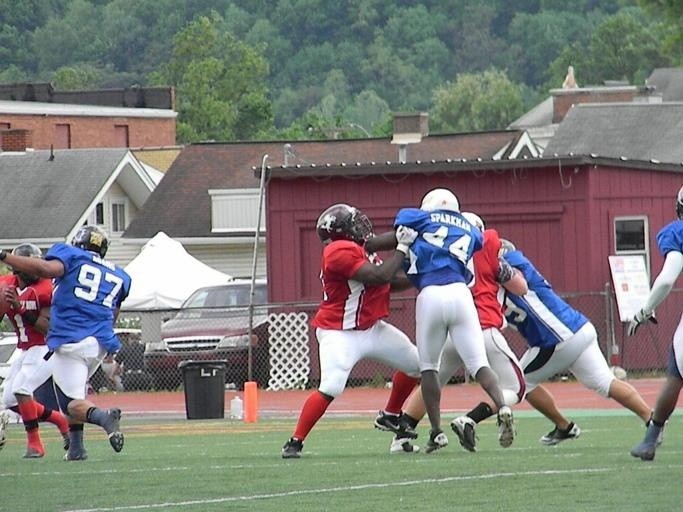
[178,358,232,420]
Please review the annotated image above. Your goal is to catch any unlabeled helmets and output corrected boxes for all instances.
[673,186,683,220]
[419,187,516,258]
[6,242,44,274]
[70,224,107,259]
[316,204,374,246]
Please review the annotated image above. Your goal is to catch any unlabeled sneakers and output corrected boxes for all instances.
[497,405,515,448]
[280,438,302,458]
[21,437,88,462]
[373,410,477,452]
[102,408,123,453]
[539,421,580,445]
[630,410,668,460]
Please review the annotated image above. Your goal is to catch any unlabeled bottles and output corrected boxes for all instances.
[230,395,243,420]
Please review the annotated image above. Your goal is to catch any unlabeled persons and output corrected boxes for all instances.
[0,243,68,460]
[390,211,528,453]
[366,189,515,453]
[108,332,148,393]
[0,224,125,461]
[497,237,670,449]
[283,202,418,460]
[626,185,683,462]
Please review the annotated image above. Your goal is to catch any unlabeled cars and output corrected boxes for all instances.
[1,327,146,394]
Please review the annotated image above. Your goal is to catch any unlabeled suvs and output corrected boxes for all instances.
[146,274,269,389]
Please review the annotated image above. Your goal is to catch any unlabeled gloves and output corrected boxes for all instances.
[626,306,659,337]
[395,225,418,256]
